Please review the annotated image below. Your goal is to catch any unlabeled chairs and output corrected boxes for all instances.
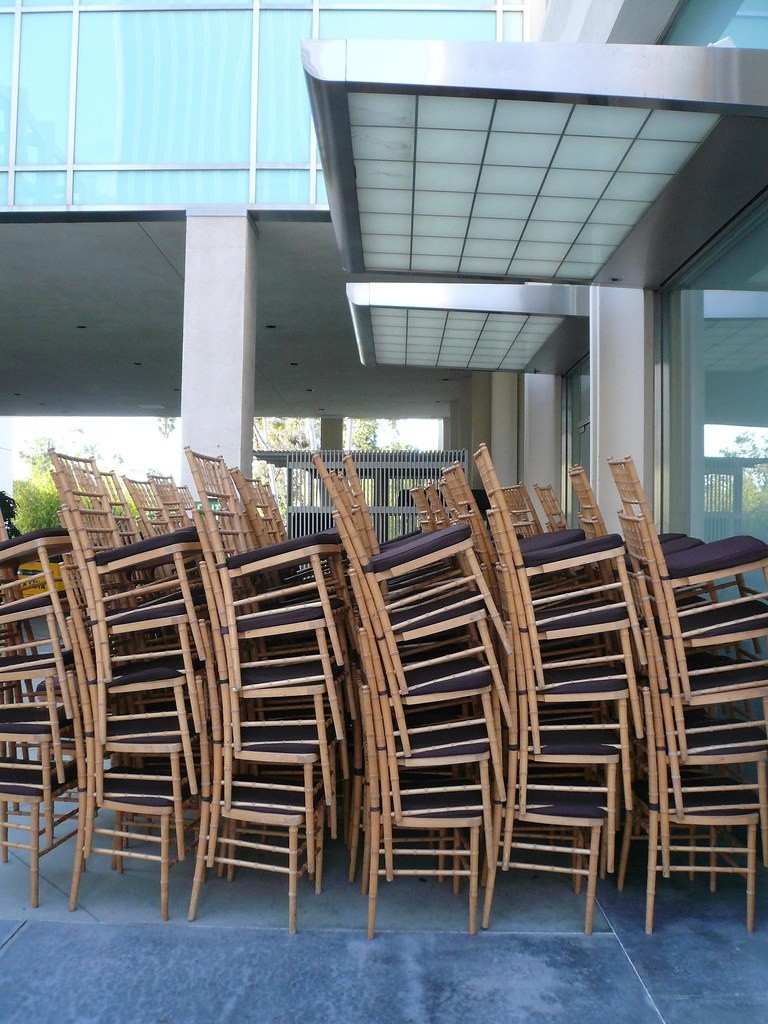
[0,444,768,935]
